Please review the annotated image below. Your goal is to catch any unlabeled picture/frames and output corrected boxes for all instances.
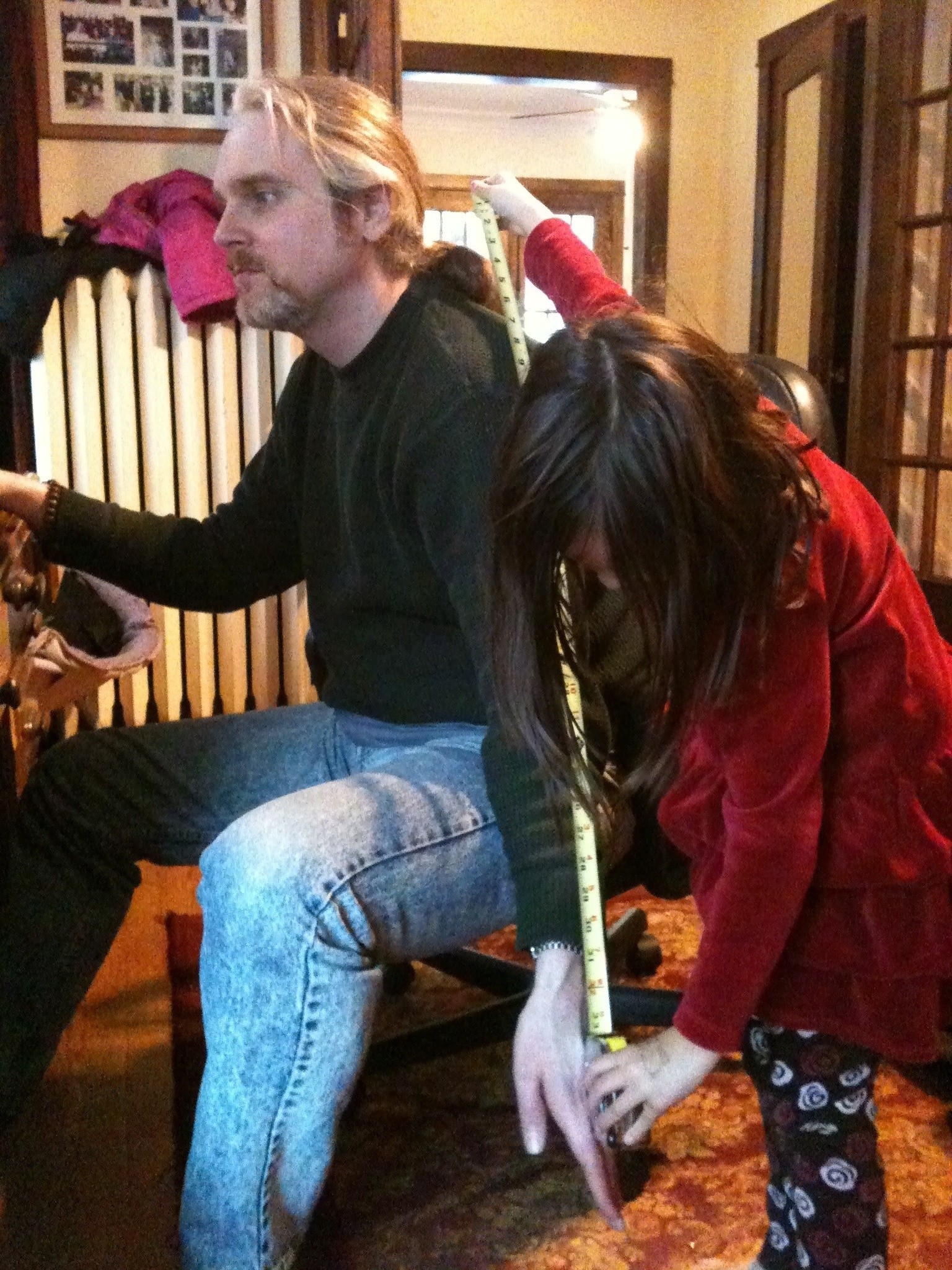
[27,0,276,145]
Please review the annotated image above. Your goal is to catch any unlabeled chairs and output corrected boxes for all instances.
[339,347,851,1199]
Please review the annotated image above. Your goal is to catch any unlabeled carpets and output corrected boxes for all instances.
[157,888,952,1270]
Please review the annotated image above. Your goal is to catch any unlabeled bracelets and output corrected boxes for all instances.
[33,481,62,546]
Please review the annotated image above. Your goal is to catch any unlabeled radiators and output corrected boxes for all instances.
[28,261,325,741]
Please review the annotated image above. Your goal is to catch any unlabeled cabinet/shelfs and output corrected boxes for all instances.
[1,503,60,802]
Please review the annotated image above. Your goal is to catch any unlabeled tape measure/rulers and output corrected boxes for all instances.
[473,185,642,1157]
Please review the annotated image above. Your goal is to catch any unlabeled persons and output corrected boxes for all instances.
[470,171,952,1270]
[60,1,248,117]
[0,72,639,1270]
